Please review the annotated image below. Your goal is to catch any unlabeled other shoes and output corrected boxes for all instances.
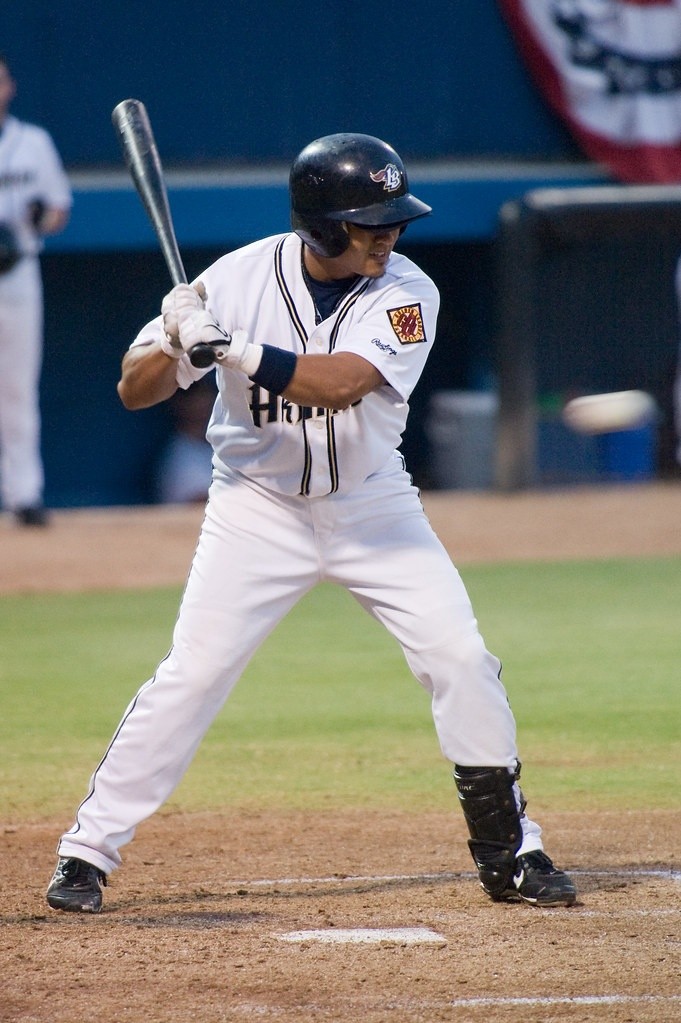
[15,505,45,525]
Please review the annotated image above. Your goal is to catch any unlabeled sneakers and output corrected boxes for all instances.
[46,855,108,913]
[500,849,578,908]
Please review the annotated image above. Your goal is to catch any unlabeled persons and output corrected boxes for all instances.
[0,64,72,527]
[47,132,579,913]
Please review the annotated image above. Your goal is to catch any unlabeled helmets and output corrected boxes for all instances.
[290,134,433,258]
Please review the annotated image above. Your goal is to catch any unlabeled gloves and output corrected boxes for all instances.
[160,280,209,359]
[175,309,264,378]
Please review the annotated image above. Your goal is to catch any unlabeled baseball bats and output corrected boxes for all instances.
[110,98,215,369]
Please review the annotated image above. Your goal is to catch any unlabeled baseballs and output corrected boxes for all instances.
[562,390,655,433]
